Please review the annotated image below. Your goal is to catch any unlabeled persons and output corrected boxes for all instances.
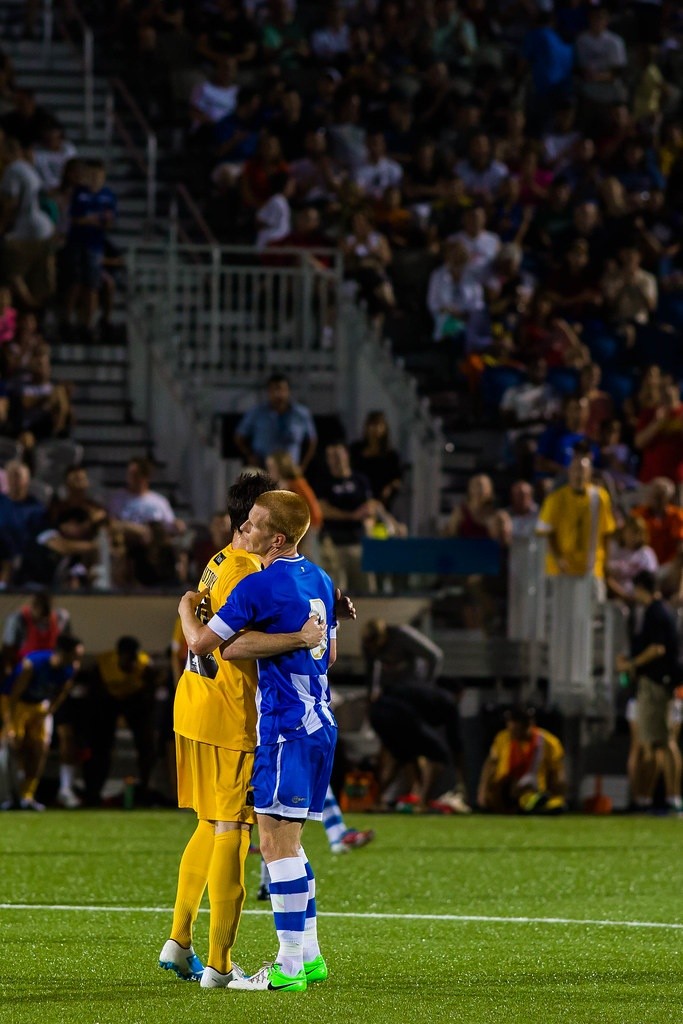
[0,0,683,816]
[176,490,342,995]
[255,846,276,903]
[321,783,376,857]
[156,468,359,992]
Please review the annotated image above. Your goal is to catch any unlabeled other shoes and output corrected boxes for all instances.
[0,798,45,812]
[397,795,431,814]
[622,798,683,817]
[439,790,473,815]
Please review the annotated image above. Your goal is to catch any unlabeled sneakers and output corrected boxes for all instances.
[330,830,374,854]
[157,938,204,982]
[199,962,251,988]
[227,962,307,993]
[304,954,328,984]
